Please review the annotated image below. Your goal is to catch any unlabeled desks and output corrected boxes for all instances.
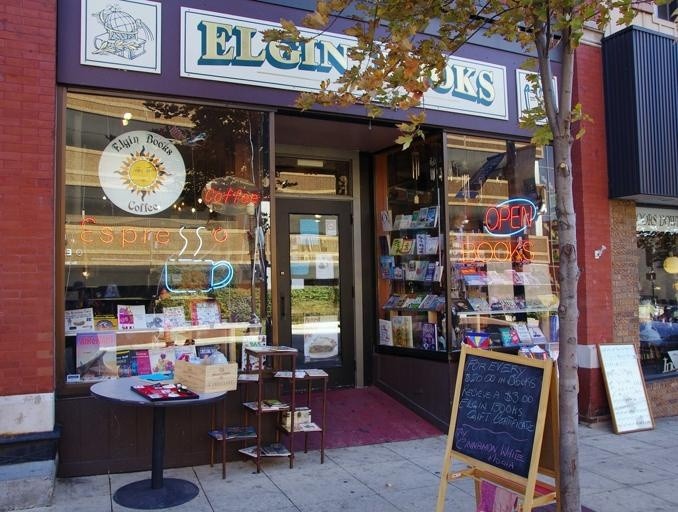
[91,372,227,508]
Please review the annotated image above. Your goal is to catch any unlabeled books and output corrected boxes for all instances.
[208,335,329,458]
[63,299,223,381]
[377,204,560,363]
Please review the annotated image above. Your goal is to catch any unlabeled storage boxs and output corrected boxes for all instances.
[174,358,238,393]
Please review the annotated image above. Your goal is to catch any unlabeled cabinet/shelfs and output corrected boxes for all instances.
[211,345,329,478]
[375,132,559,356]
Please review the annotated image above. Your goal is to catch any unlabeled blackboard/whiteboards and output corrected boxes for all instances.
[446,345,554,486]
[596,341,656,434]
